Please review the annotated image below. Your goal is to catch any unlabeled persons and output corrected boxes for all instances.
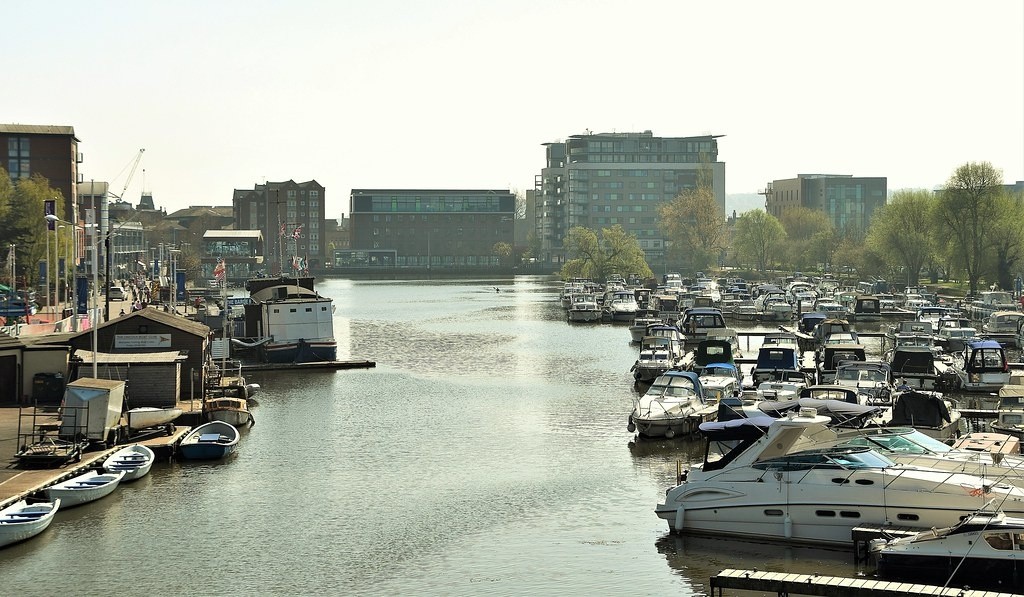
[119,268,150,317]
[102,305,106,323]
[194,297,201,314]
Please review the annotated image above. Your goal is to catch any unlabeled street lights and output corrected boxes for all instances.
[169,250,180,315]
[91,179,156,379]
[158,243,164,289]
[111,234,121,281]
[151,248,156,281]
[44,215,87,278]
[174,228,178,250]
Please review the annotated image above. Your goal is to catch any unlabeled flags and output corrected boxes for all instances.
[214,258,226,284]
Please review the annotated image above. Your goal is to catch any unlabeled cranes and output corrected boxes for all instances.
[108,148,145,204]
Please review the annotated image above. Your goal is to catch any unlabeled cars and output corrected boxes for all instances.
[207,280,217,287]
[108,287,128,301]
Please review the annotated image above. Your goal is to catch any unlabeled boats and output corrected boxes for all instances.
[204,360,260,426]
[41,470,126,509]
[559,271,1024,594]
[180,420,240,460]
[127,404,183,430]
[0,498,61,547]
[165,189,338,364]
[103,443,155,482]
